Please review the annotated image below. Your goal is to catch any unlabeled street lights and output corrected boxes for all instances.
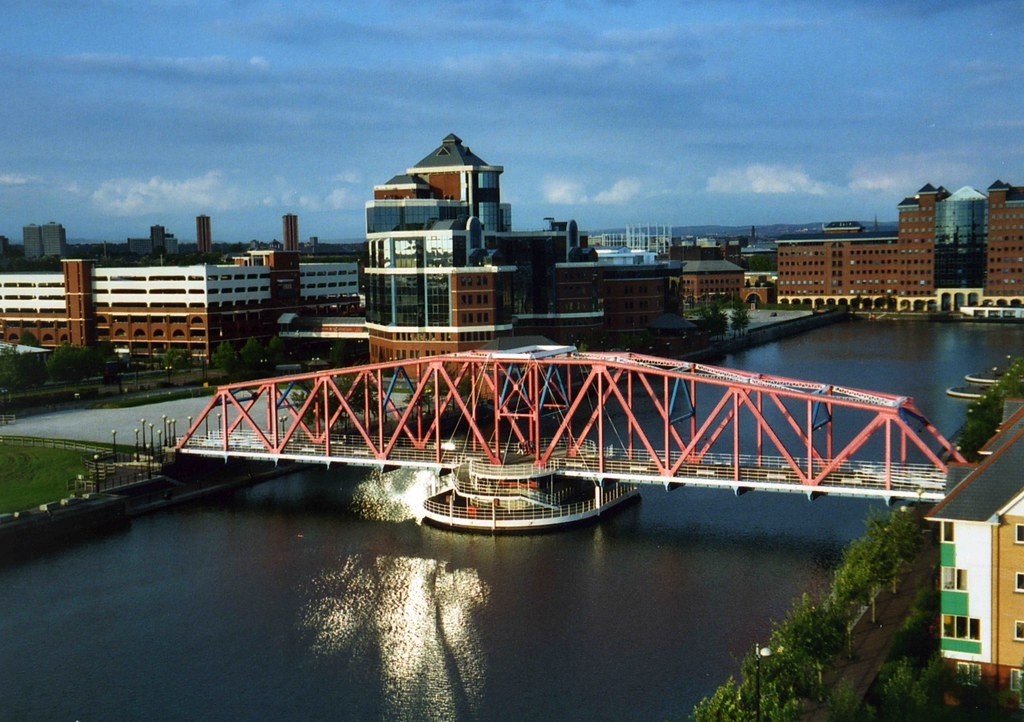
[162,414,167,447]
[145,443,151,479]
[140,418,147,454]
[188,416,192,429]
[279,416,288,438]
[112,429,118,463]
[133,428,141,461]
[165,366,172,385]
[217,413,222,437]
[260,359,268,378]
[149,423,155,456]
[172,418,176,445]
[205,414,209,439]
[157,430,164,462]
[93,454,100,494]
[755,641,773,722]
[166,420,172,448]
